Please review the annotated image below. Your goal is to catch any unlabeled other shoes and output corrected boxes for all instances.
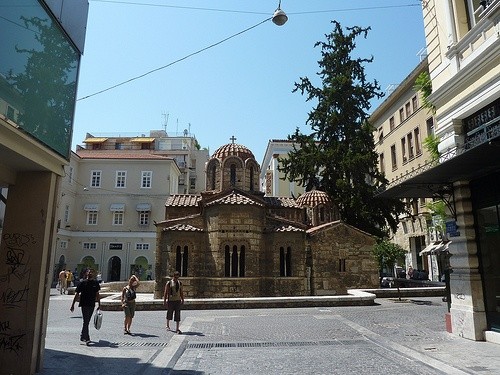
[61,292,65,294]
[166,326,170,331]
[123,327,131,335]
[175,329,181,334]
[79,338,92,346]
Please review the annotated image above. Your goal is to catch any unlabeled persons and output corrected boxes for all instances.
[58,267,103,295]
[145,267,153,281]
[121,275,141,336]
[164,271,184,334]
[70,267,102,346]
[168,265,176,277]
[381,268,431,288]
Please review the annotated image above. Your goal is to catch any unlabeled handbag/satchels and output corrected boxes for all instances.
[93,309,103,330]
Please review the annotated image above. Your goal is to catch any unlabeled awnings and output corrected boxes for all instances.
[362,133,500,209]
[419,241,453,256]
[82,138,108,143]
[130,138,155,143]
